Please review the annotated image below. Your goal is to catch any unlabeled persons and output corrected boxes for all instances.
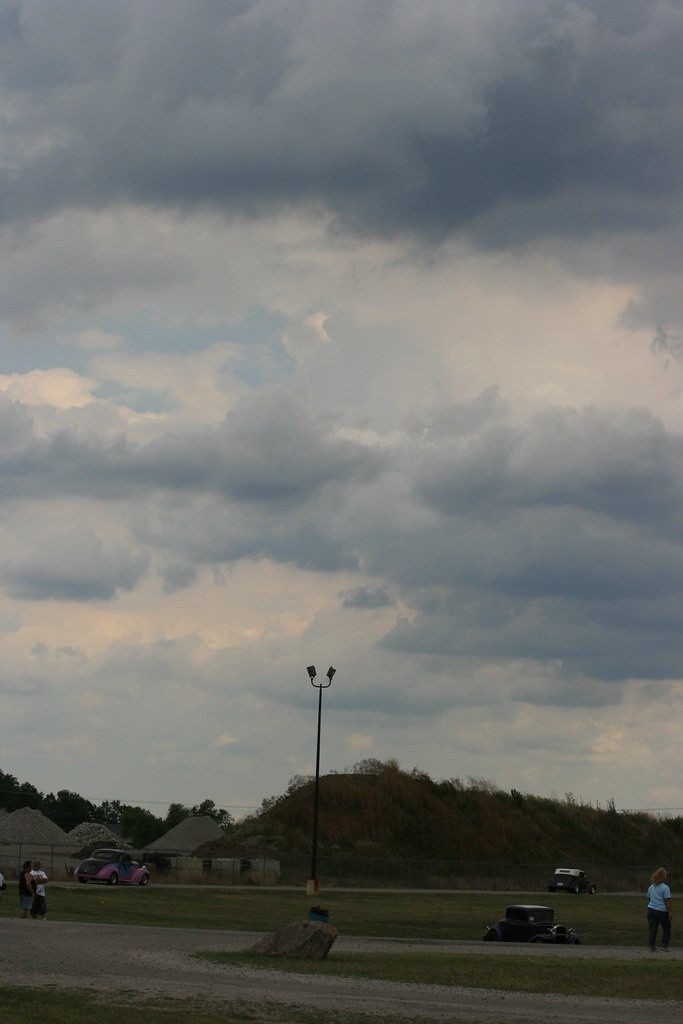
[29,861,49,920]
[647,867,672,952]
[18,861,37,919]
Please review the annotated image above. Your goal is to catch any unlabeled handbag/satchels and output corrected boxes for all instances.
[0,876,6,891]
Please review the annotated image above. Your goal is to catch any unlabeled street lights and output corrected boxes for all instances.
[306,665,337,879]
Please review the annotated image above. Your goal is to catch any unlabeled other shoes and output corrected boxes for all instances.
[660,948,671,952]
[649,945,656,952]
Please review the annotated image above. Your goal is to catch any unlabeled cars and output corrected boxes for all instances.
[547,868,597,895]
[74,848,151,886]
[480,904,582,945]
